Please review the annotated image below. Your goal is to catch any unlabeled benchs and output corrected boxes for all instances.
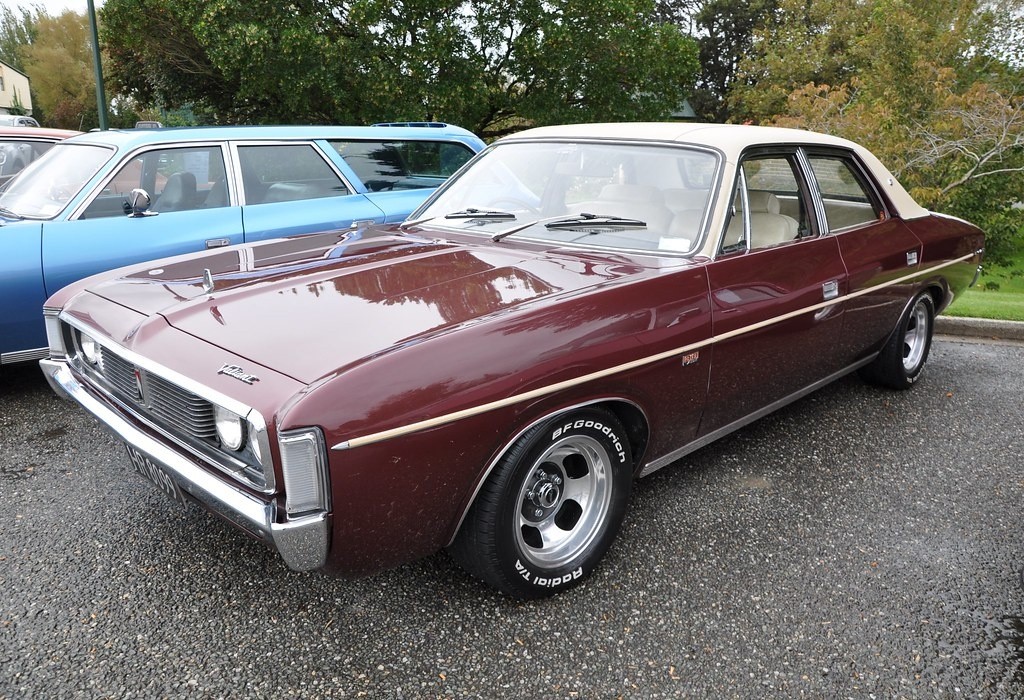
[260,181,347,204]
[776,195,876,230]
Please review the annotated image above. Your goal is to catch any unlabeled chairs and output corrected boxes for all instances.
[666,188,800,250]
[571,183,671,233]
[150,172,197,214]
[198,174,228,209]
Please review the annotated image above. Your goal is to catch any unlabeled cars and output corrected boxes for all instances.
[38,122,987,602]
[0,121,543,365]
[1,126,215,203]
